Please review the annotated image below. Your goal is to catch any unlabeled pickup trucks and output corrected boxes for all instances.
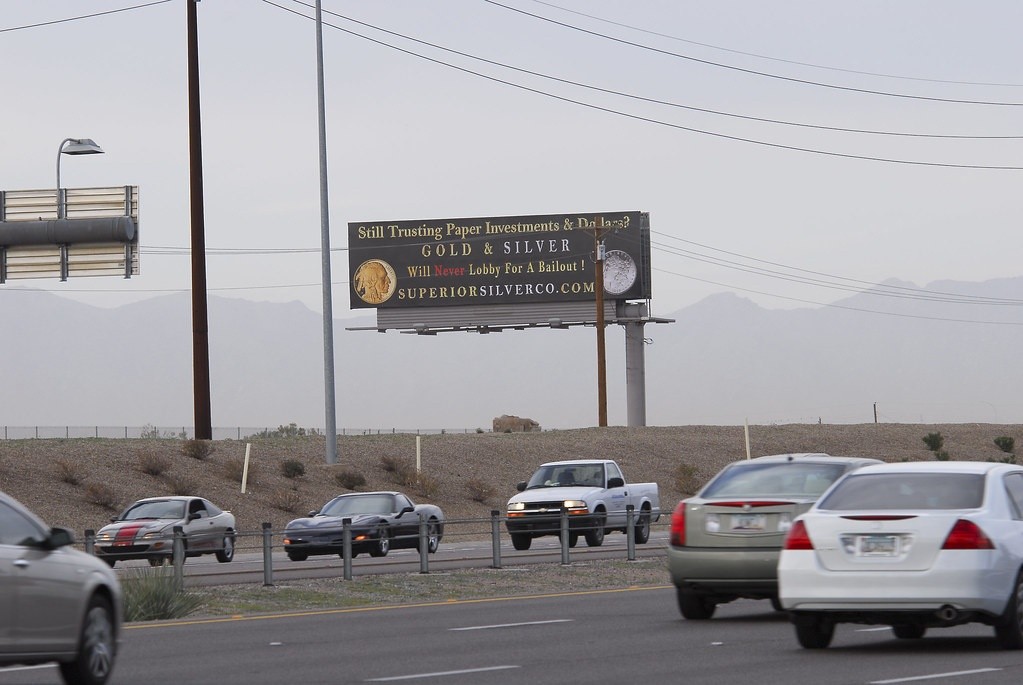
[504,457,662,550]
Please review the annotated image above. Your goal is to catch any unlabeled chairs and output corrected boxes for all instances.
[804,479,831,495]
[558,472,574,485]
[737,477,781,495]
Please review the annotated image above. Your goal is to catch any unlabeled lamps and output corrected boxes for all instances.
[56,137,106,218]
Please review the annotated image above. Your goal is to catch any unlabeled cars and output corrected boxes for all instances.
[664,453,889,620]
[0,492,124,684]
[774,459,1022,654]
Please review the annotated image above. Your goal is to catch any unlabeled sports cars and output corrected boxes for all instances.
[282,489,448,561]
[92,495,238,569]
